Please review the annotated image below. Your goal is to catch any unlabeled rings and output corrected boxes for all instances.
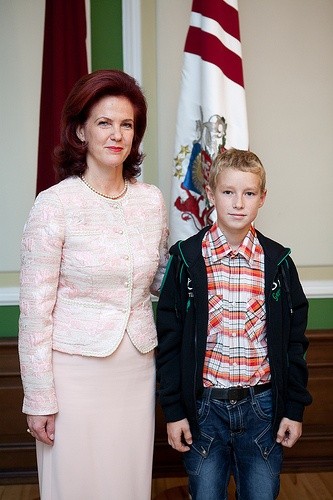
[26,428,32,433]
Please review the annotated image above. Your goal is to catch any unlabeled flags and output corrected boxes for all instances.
[157,0,249,246]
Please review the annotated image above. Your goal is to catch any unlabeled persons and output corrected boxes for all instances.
[15,69,170,500]
[155,147,314,500]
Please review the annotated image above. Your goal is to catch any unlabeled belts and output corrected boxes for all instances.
[197,382,272,403]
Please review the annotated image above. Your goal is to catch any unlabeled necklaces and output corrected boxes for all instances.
[78,170,129,199]
